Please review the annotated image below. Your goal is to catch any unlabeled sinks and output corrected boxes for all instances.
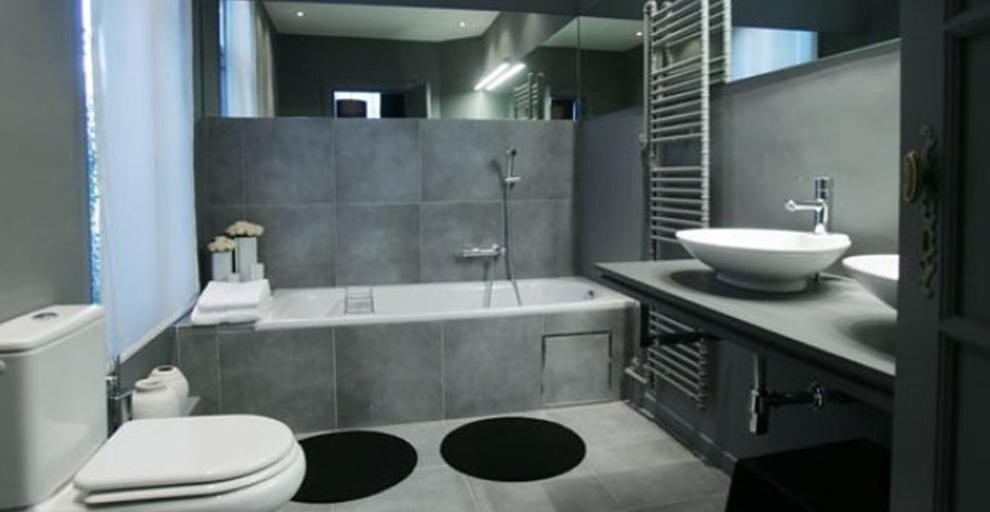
[841,253,900,311]
[675,227,852,293]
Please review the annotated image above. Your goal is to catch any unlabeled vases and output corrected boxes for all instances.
[210,236,257,280]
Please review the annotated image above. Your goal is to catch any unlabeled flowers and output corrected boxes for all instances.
[207,218,262,253]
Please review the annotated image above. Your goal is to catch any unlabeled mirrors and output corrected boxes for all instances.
[221,3,652,120]
[724,1,902,86]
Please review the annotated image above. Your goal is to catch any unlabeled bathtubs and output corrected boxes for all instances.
[170,276,643,433]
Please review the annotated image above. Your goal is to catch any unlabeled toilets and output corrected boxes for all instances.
[1,303,306,512]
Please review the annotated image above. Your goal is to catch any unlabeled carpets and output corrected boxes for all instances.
[290,416,585,502]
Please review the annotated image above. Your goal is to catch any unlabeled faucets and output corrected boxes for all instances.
[489,242,506,261]
[781,176,830,231]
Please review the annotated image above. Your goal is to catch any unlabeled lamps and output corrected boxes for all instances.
[470,58,527,90]
